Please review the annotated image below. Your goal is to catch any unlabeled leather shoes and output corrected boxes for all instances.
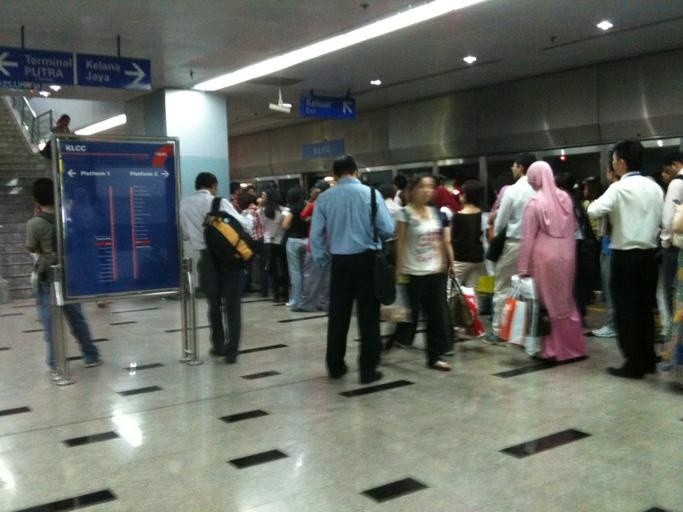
[428,360,452,370]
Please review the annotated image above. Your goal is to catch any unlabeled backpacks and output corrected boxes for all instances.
[202,196,256,269]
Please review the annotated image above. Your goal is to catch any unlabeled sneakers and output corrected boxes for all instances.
[590,324,670,378]
[480,333,507,345]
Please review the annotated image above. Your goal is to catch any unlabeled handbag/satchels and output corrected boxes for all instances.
[364,251,395,304]
[447,274,551,356]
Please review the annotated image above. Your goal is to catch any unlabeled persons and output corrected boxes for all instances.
[483,150,600,362]
[231,177,330,312]
[176,171,255,365]
[22,179,104,376]
[53,114,73,137]
[311,156,396,384]
[366,175,484,370]
[583,137,683,394]
